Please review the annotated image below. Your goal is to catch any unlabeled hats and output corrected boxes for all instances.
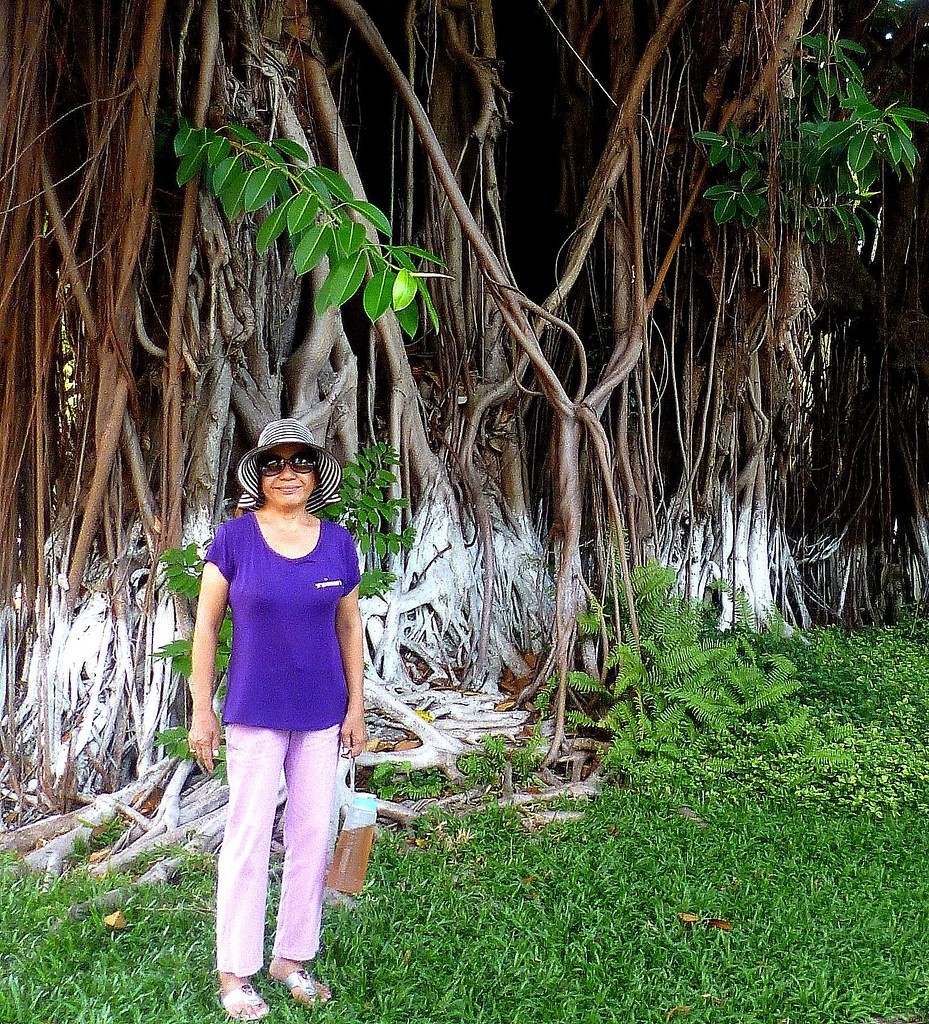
[235,418,342,514]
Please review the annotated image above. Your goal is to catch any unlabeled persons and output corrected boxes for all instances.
[184,419,370,1022]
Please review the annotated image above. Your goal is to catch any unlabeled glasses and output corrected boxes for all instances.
[257,450,317,476]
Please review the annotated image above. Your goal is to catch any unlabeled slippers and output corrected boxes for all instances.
[271,968,331,1008]
[217,982,270,1021]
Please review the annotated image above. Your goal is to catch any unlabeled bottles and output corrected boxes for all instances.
[328,792,378,893]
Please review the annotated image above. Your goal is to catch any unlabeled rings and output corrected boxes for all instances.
[189,747,196,753]
